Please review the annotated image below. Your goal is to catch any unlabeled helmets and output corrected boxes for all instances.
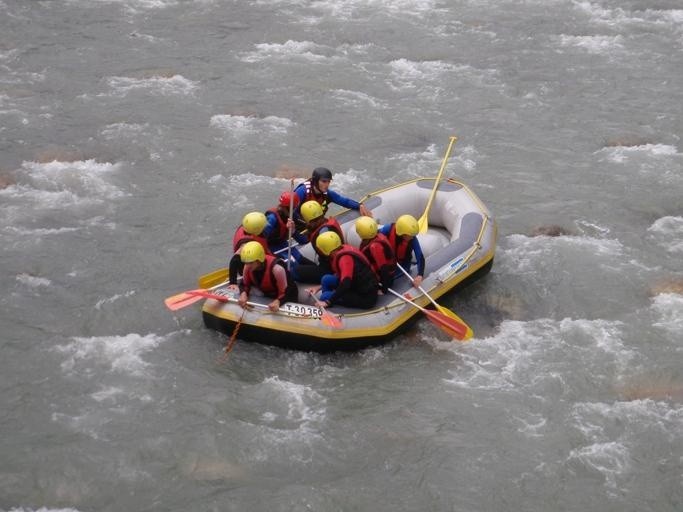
[240,168,419,263]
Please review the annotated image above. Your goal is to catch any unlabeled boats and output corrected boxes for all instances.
[201,177,498,358]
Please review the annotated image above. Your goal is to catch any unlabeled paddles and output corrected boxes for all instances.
[163,276,243,311]
[304,289,341,328]
[378,283,467,340]
[417,136,457,234]
[184,291,311,318]
[395,262,473,341]
[197,243,299,288]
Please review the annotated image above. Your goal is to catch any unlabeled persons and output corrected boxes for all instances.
[227,167,425,311]
